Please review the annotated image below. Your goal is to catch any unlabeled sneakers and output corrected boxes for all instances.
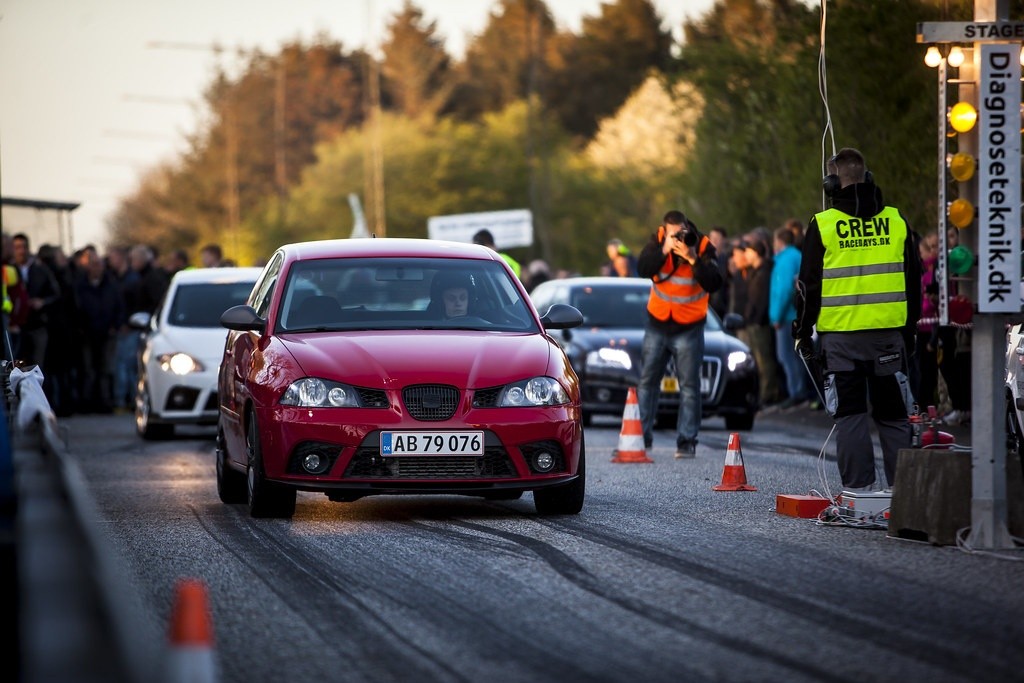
[675,435,698,458]
[612,435,653,455]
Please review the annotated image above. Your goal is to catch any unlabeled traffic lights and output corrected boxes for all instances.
[938,57,978,329]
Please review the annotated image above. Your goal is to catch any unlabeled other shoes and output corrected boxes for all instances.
[779,396,810,415]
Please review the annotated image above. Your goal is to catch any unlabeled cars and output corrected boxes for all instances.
[130,266,324,440]
[217,237,587,521]
[526,276,762,433]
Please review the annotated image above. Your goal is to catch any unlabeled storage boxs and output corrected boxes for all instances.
[842,490,892,516]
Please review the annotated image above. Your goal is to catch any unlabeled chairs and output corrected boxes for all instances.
[288,296,345,325]
[427,294,483,318]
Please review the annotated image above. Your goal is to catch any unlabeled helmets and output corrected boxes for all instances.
[429,271,478,318]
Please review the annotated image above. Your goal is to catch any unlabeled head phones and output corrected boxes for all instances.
[822,154,874,197]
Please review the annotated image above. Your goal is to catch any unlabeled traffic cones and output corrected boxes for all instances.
[713,433,758,491]
[152,582,221,683]
[610,387,656,464]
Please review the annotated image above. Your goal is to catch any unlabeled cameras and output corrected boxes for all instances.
[671,227,697,246]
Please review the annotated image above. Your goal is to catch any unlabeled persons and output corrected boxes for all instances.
[525,259,550,293]
[430,268,479,320]
[0,230,236,419]
[789,148,924,492]
[473,229,521,281]
[708,220,825,411]
[602,238,638,278]
[902,223,971,424]
[612,209,723,457]
